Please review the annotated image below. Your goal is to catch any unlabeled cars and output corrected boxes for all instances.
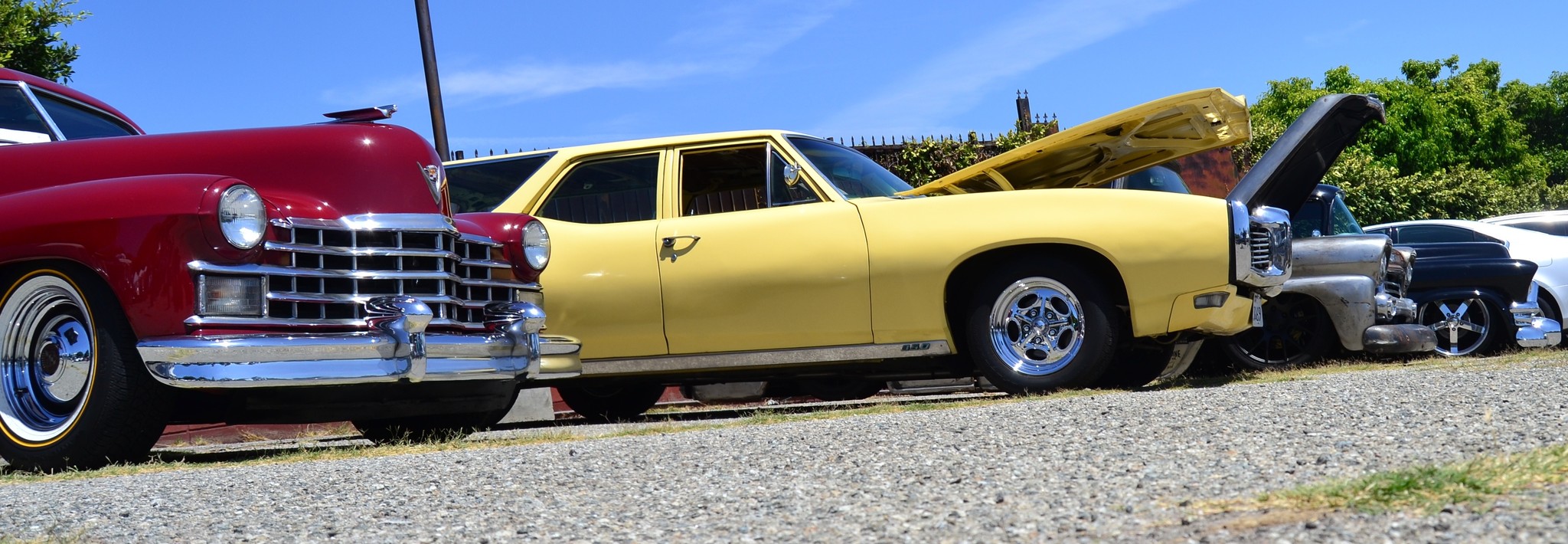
[1107,93,1568,371]
[440,86,1294,422]
[0,67,584,474]
